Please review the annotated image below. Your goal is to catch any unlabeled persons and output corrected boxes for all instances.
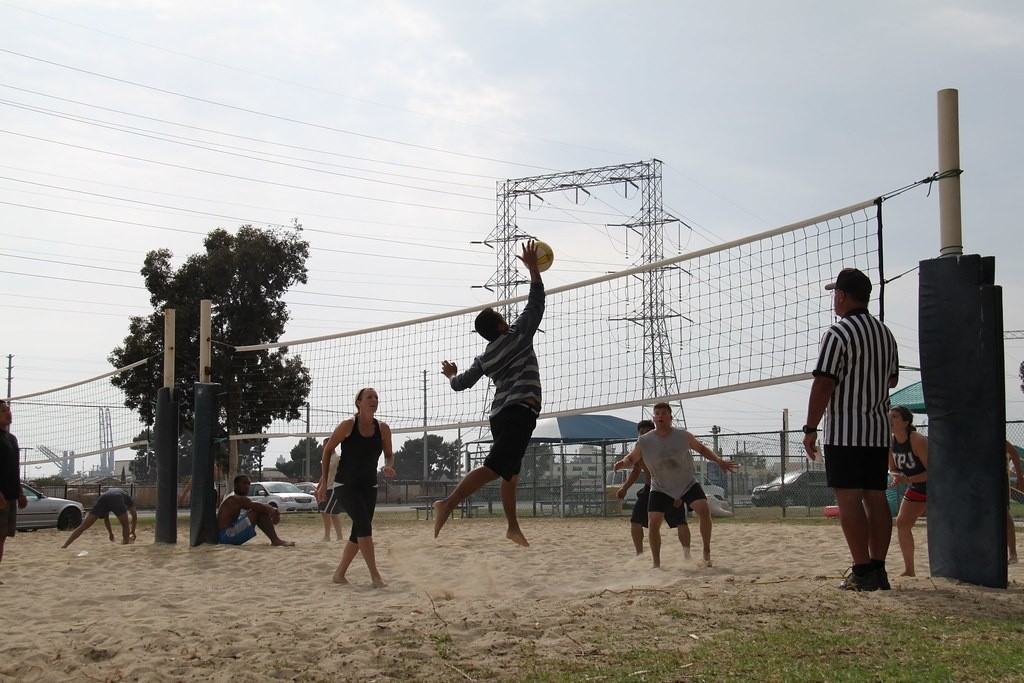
[61,488,137,548]
[804,268,898,591]
[0,401,27,584]
[612,402,739,567]
[316,438,343,542]
[318,388,394,586]
[434,240,546,546]
[179,459,218,507]
[217,475,295,546]
[617,421,692,560]
[889,407,928,577]
[1005,441,1024,564]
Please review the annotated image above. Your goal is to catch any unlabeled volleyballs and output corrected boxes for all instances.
[122,536,134,544]
[522,241,554,272]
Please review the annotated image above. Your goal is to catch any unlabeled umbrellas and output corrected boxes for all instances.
[888,380,926,414]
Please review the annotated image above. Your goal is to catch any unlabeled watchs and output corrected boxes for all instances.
[803,426,816,433]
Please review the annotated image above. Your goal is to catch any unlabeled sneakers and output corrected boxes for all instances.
[870,558,890,590]
[839,565,878,591]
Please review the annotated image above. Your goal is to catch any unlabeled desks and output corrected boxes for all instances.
[416,495,473,519]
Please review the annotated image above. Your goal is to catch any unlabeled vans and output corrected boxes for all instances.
[603,468,726,510]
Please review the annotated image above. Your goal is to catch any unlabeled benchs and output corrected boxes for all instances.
[545,490,609,516]
[410,505,484,519]
[535,498,636,516]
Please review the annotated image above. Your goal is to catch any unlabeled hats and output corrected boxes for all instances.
[825,268,871,299]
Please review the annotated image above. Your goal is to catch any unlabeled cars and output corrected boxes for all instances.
[219,482,318,513]
[287,482,319,496]
[15,482,86,533]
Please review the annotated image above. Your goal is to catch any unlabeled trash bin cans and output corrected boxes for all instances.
[606,485,623,514]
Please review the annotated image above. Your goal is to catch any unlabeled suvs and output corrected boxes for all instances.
[751,470,836,508]
